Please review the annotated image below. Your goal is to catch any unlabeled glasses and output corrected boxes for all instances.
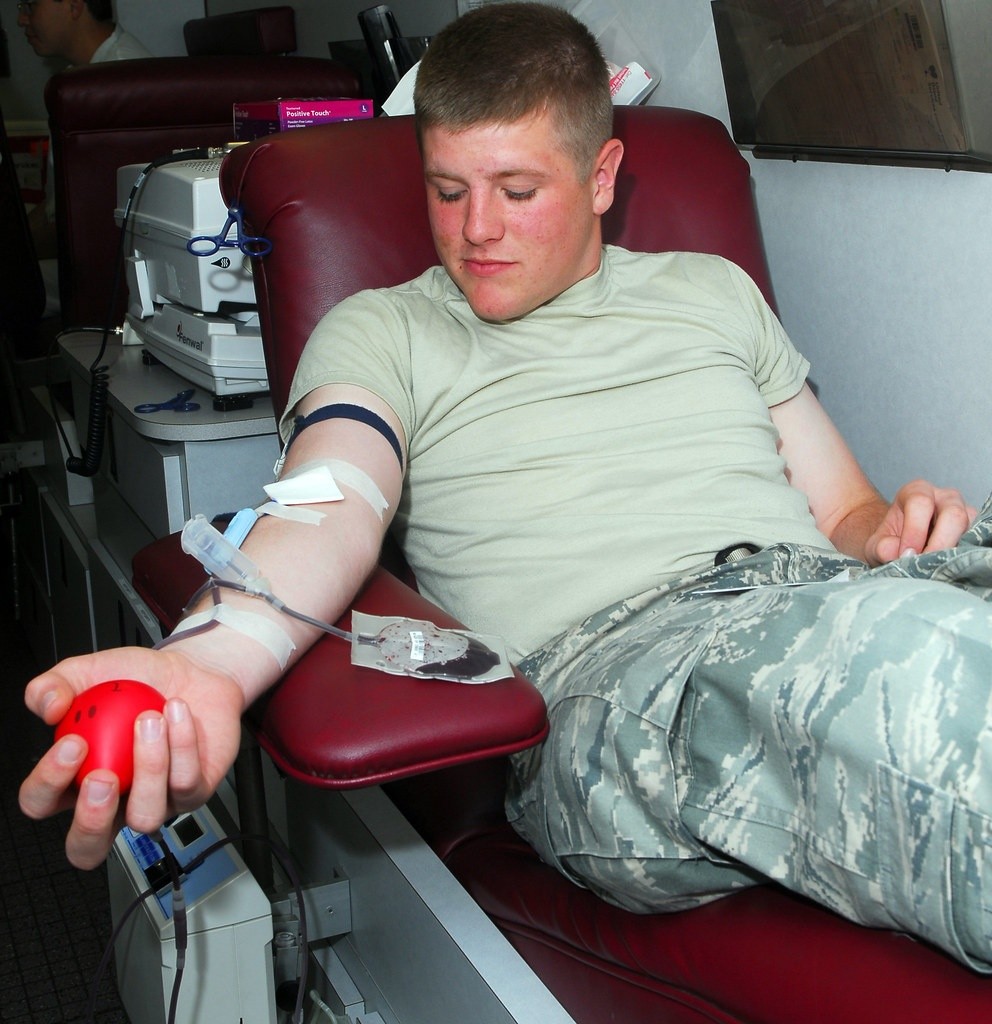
[16,0,38,16]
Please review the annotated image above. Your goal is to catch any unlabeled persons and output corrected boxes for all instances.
[17,2,992,1007]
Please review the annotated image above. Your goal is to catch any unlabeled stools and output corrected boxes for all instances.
[42,53,373,344]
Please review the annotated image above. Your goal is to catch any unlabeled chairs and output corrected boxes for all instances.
[127,104,992,1024]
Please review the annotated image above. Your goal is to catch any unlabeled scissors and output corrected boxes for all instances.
[186,205,273,257]
[133,388,200,415]
